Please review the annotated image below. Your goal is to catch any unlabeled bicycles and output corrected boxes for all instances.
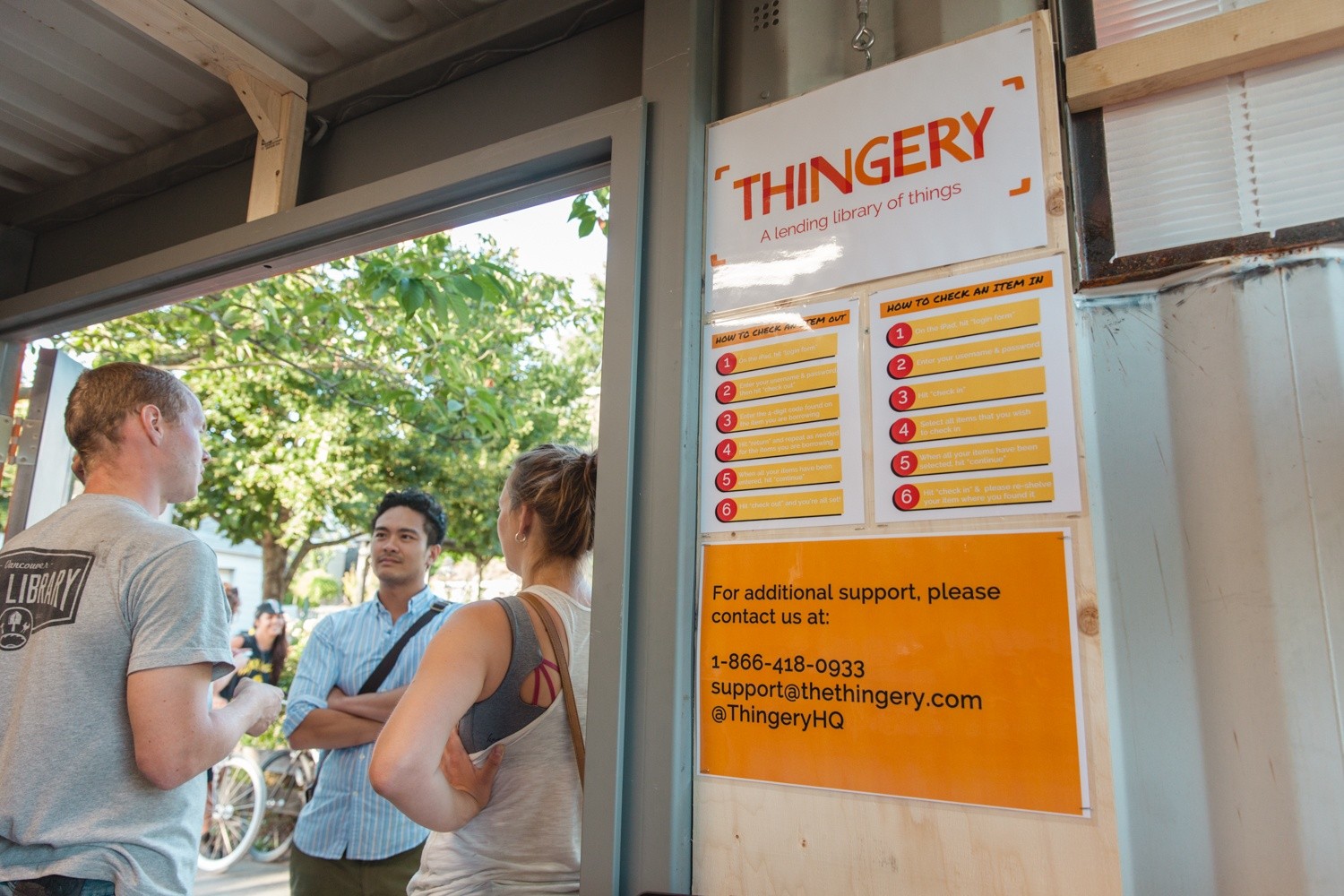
[195,744,321,874]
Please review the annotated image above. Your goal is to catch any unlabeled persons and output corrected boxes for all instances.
[369,443,598,896]
[284,489,465,896]
[0,363,284,896]
[201,599,288,844]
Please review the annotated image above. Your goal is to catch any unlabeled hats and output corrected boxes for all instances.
[257,599,283,615]
